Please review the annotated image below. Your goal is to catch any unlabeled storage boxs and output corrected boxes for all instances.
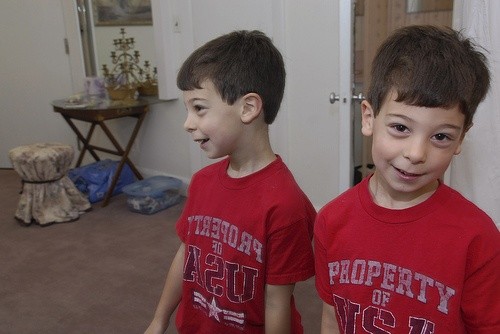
[121,176,182,215]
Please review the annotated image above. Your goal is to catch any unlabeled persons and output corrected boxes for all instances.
[312,24,500,334]
[142,30,318,334]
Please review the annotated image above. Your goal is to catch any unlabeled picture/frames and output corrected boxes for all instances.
[91,0,152,27]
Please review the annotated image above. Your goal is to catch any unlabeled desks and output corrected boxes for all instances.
[50,96,150,207]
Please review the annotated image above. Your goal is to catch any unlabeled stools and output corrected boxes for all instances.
[10,143,92,225]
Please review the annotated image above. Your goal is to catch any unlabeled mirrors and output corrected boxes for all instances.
[0,0,500,334]
